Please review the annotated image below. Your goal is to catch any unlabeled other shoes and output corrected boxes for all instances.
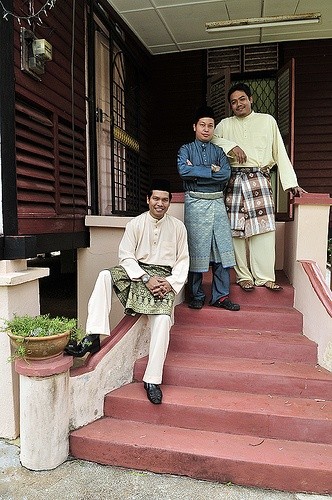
[214,298,240,311]
[189,299,204,309]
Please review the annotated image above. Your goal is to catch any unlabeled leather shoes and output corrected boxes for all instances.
[64,335,100,357]
[144,382,162,404]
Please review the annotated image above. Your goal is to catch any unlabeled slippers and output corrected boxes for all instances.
[265,282,283,291]
[239,281,255,291]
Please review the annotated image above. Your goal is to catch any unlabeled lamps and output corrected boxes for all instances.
[205,12,321,32]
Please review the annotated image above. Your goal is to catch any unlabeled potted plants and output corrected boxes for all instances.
[0,313,92,368]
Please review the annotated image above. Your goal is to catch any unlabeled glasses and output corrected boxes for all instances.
[231,96,248,105]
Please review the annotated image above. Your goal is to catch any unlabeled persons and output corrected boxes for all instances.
[64,185,190,404]
[210,83,309,292]
[177,107,240,311]
